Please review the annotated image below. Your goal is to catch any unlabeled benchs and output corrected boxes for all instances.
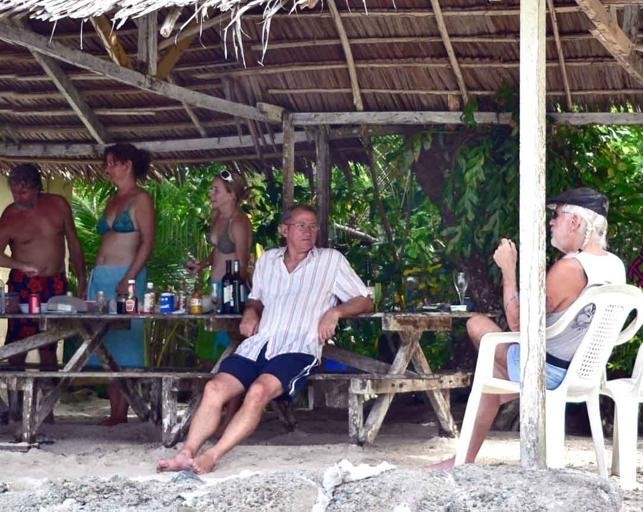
[0,369,474,444]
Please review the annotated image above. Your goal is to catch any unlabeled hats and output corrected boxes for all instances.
[545,187,609,219]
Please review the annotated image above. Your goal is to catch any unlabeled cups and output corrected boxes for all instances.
[452,269,470,305]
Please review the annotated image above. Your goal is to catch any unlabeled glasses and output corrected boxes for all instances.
[548,208,568,220]
[283,223,321,232]
[218,169,234,184]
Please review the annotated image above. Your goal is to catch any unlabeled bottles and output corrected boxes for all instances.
[221,260,234,314]
[117,293,128,313]
[126,279,138,313]
[190,283,203,315]
[159,292,176,314]
[363,261,376,314]
[0,279,6,315]
[144,282,156,313]
[28,295,41,313]
[96,290,107,313]
[229,260,245,314]
[212,282,221,314]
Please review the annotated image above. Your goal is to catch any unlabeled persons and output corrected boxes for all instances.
[183,170,254,440]
[426,186,627,470]
[1,162,87,426]
[156,205,375,473]
[83,144,156,425]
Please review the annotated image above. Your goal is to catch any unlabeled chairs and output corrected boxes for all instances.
[600,341,643,479]
[453,283,643,479]
